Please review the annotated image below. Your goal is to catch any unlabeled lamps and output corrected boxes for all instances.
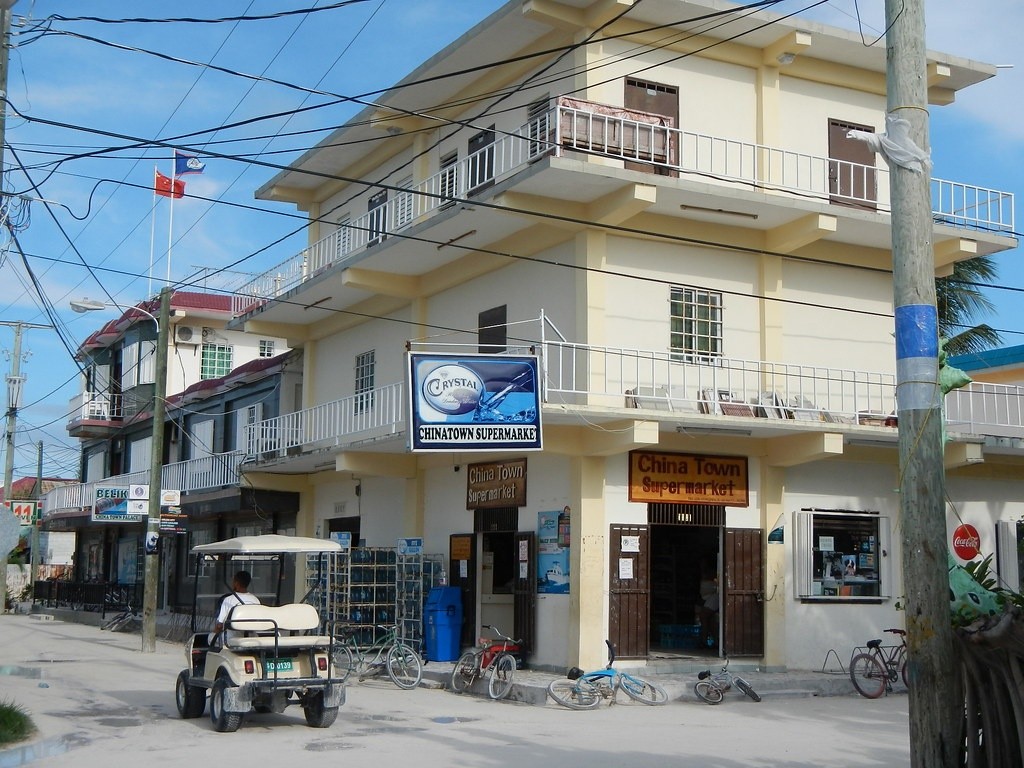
[675,427,752,437]
[437,230,476,251]
[385,126,403,135]
[680,205,758,220]
[304,297,332,311]
[315,460,336,470]
[777,51,796,65]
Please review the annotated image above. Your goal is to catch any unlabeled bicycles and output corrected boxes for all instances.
[694,648,760,705]
[48,573,134,612]
[849,627,908,700]
[450,624,522,700]
[548,639,668,710]
[333,621,429,688]
[100,599,138,633]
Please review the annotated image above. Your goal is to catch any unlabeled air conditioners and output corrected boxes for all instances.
[174,324,202,346]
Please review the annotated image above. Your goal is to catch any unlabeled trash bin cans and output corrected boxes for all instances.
[423,586,463,661]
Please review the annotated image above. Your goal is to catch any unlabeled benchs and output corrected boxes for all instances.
[209,603,336,654]
[538,93,675,177]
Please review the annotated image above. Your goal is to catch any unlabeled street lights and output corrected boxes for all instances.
[70,300,168,654]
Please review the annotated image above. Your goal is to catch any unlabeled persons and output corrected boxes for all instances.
[208,571,261,651]
[698,576,719,648]
[844,560,855,576]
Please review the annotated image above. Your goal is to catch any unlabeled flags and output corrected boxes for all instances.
[175,152,206,175]
[155,171,186,199]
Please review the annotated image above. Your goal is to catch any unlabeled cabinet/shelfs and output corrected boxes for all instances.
[305,546,443,662]
